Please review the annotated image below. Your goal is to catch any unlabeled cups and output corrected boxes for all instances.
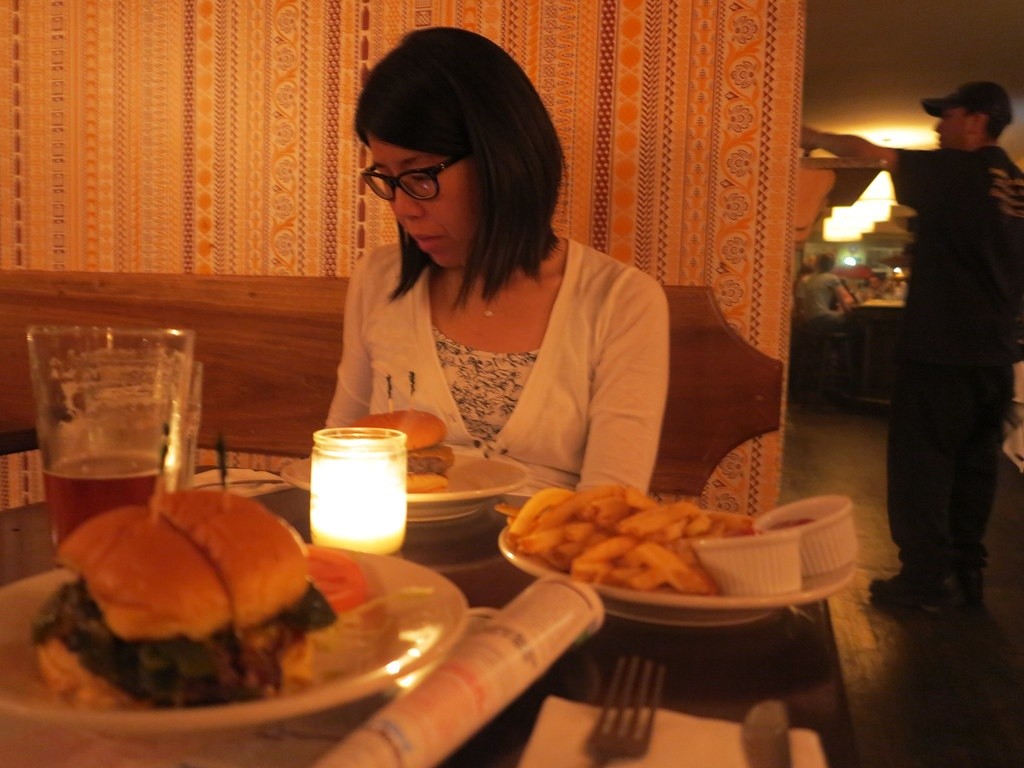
[81,350,203,494]
[26,323,193,546]
[753,496,856,586]
[692,532,802,597]
[308,428,407,556]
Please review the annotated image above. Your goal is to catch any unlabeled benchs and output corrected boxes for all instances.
[0,267,783,499]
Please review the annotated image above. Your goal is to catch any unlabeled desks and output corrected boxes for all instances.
[0,500,858,768]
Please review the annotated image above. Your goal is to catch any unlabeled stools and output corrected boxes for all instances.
[794,331,850,414]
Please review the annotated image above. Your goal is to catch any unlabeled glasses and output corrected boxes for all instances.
[360,154,471,200]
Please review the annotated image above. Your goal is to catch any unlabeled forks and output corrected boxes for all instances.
[586,657,665,768]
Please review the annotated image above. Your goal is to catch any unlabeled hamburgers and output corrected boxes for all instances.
[29,486,337,708]
[349,408,457,493]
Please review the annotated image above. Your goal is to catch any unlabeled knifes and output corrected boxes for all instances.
[742,700,791,768]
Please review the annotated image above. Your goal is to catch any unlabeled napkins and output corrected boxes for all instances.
[516,694,830,768]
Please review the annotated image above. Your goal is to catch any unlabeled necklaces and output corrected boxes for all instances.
[484,310,493,317]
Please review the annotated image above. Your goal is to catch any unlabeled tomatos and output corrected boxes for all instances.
[304,546,372,614]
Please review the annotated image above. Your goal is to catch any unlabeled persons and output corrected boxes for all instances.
[324,27,671,496]
[796,252,854,332]
[799,81,1024,612]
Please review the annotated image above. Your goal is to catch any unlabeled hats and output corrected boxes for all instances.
[921,80,1012,126]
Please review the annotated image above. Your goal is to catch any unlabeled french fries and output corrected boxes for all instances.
[493,478,761,599]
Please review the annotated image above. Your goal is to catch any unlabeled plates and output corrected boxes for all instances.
[0,547,468,735]
[498,507,856,627]
[282,454,524,521]
